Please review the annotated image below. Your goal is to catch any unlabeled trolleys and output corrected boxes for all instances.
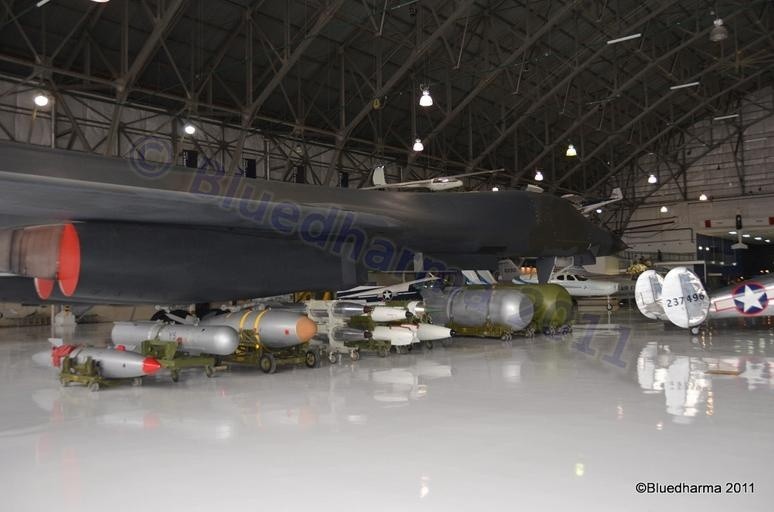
[450,317,573,341]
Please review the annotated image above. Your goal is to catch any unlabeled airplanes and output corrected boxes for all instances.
[634,266,774,334]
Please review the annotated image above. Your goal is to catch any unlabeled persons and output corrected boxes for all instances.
[657,249,663,261]
[633,255,644,264]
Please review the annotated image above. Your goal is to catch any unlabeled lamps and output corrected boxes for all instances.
[0,0,774,215]
